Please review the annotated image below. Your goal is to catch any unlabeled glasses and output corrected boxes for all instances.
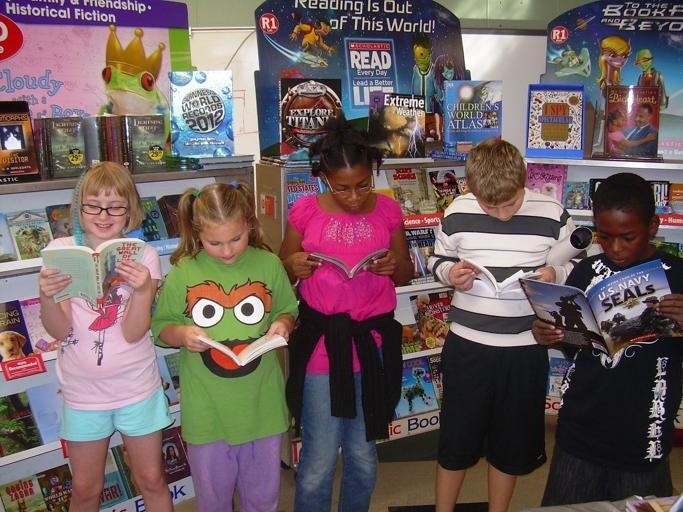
[320,168,375,199]
[81,204,129,217]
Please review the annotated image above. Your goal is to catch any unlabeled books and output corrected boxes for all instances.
[520,259,682,359]
[463,257,544,298]
[522,162,683,224]
[261,79,502,165]
[40,238,150,309]
[1,424,192,511]
[395,354,443,418]
[526,84,664,161]
[2,295,58,368]
[548,355,575,397]
[372,169,468,215]
[156,351,183,409]
[1,380,62,455]
[308,249,389,279]
[395,291,454,354]
[405,227,445,285]
[1,194,183,265]
[284,172,327,206]
[0,69,234,184]
[195,333,288,366]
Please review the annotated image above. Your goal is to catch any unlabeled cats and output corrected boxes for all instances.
[1,126,25,150]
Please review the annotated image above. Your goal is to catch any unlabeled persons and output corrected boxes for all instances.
[532,173,682,507]
[428,136,586,511]
[40,162,176,511]
[151,182,299,511]
[279,129,416,511]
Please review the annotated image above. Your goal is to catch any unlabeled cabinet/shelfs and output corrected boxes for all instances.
[525,158,683,418]
[256,148,470,469]
[1,168,256,512]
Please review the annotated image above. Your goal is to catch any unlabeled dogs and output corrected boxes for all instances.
[0,330,27,365]
